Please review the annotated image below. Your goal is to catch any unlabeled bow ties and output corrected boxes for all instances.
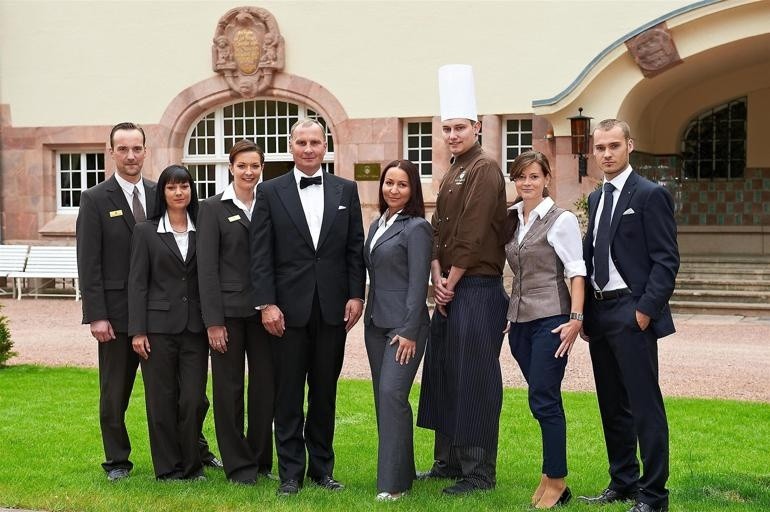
[299,175,323,189]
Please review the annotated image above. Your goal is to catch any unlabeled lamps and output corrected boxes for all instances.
[566,107,595,183]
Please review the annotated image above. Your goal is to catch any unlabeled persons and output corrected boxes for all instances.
[193,138,281,487]
[127,164,212,485]
[581,117,681,510]
[259,33,279,64]
[214,36,235,65]
[75,122,224,482]
[416,116,513,497]
[248,119,369,498]
[502,149,589,512]
[361,158,436,504]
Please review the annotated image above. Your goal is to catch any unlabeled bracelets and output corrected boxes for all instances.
[569,312,584,321]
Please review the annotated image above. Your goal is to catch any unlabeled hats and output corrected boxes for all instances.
[438,64,479,123]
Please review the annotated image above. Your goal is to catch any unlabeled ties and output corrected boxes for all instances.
[132,186,146,223]
[594,182,617,290]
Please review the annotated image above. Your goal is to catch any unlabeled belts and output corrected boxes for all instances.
[590,286,633,300]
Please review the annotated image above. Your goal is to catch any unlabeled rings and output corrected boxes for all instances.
[216,342,221,346]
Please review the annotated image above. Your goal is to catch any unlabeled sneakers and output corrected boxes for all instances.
[209,457,223,468]
[375,489,407,502]
[533,487,571,509]
[105,466,128,481]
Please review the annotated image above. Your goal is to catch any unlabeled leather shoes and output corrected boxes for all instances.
[316,476,344,491]
[577,488,640,504]
[627,501,668,512]
[276,478,304,495]
[415,468,441,480]
[443,479,481,494]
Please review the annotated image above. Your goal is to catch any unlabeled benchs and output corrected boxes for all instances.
[0,245,31,298]
[8,246,82,302]
